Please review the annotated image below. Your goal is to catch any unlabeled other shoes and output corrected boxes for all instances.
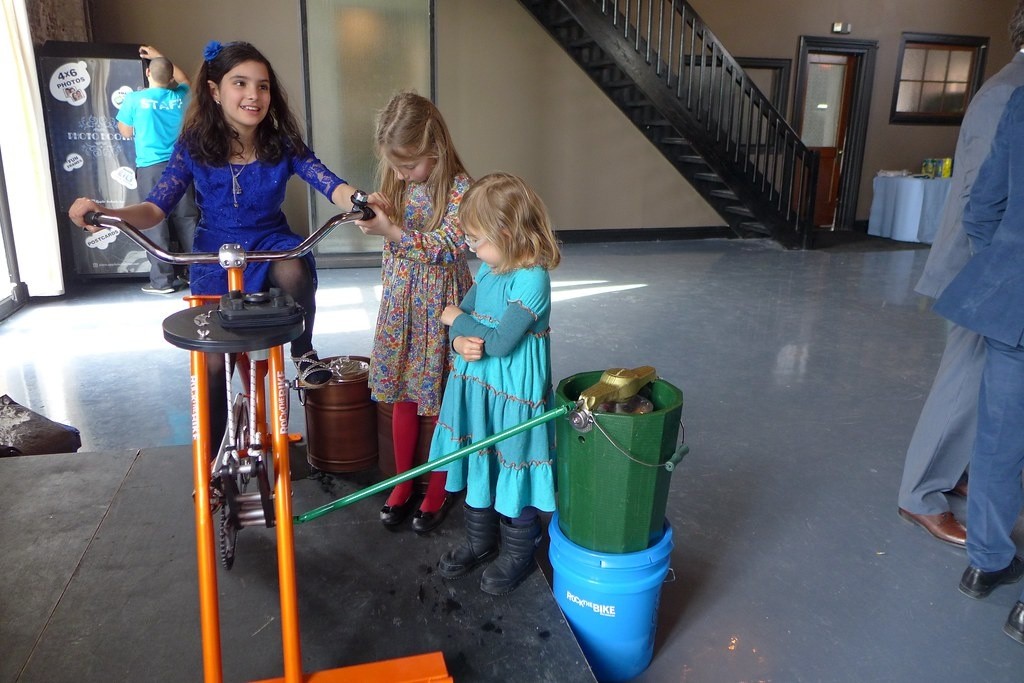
[177,274,190,284]
[141,283,174,293]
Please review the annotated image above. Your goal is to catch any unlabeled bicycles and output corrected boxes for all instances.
[76,195,378,572]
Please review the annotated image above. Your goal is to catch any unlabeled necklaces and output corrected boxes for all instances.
[226,143,256,208]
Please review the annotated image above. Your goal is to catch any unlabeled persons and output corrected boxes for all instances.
[116,47,195,293]
[65,88,82,100]
[897,0,1024,545]
[352,94,476,534]
[932,87,1023,643]
[69,41,394,513]
[428,174,562,592]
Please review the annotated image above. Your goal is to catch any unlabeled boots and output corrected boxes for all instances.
[479,513,543,596]
[437,502,500,580]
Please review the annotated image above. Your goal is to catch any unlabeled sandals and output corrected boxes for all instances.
[290,350,333,389]
[193,482,223,515]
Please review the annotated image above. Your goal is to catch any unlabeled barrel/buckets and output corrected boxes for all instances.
[553,371,689,553]
[921,158,952,178]
[374,400,436,495]
[549,508,677,683]
[302,355,378,474]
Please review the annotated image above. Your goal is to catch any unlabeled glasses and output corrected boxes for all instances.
[464,234,486,252]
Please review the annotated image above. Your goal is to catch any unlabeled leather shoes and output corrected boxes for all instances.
[410,490,454,533]
[1001,601,1024,644]
[379,484,417,525]
[959,553,1024,600]
[896,506,968,546]
[946,470,968,498]
